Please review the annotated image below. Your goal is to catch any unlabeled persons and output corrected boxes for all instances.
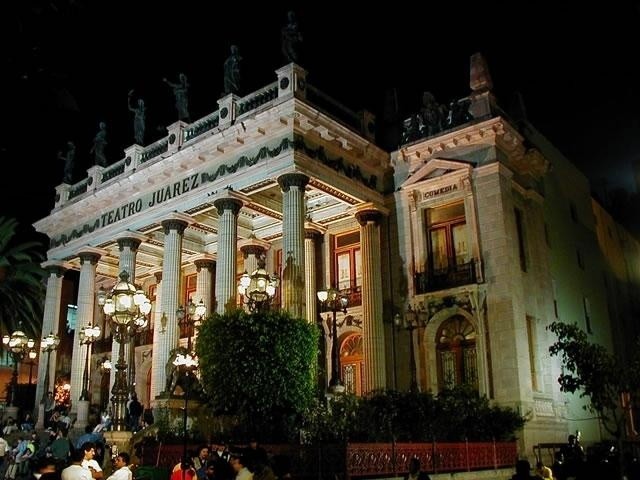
[404,458,431,480]
[515,434,640,480]
[0,387,276,479]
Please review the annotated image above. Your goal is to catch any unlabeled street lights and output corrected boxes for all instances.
[2,331,37,416]
[79,322,100,400]
[101,359,112,404]
[394,304,429,397]
[103,270,152,433]
[172,297,207,441]
[238,256,280,317]
[317,288,349,401]
[41,331,61,402]
